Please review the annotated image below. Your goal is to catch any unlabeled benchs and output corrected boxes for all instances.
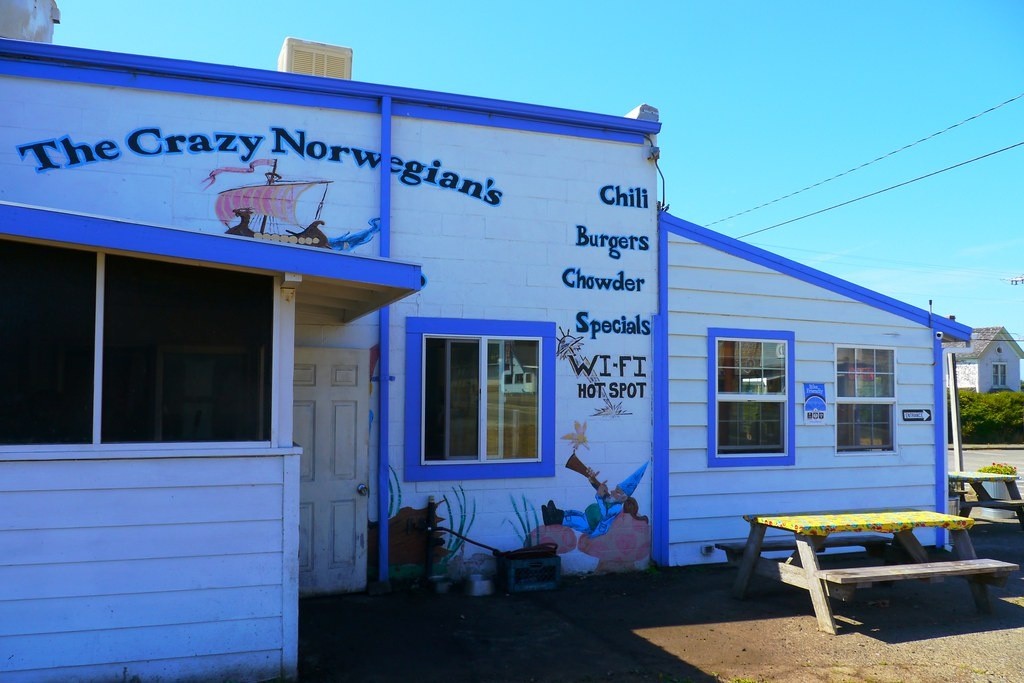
[960,499,1024,512]
[812,559,1019,602]
[714,535,893,567]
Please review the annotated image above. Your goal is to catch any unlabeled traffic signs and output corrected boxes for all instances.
[902,408,934,424]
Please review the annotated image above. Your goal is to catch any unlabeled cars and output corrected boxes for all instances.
[503,371,535,394]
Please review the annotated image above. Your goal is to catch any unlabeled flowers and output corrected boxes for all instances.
[975,462,1019,476]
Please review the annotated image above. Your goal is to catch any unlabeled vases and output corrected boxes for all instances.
[979,481,1016,519]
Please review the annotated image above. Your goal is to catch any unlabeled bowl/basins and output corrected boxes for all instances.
[467,575,492,595]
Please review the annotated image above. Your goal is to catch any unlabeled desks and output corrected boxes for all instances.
[732,510,992,636]
[946,471,1024,525]
